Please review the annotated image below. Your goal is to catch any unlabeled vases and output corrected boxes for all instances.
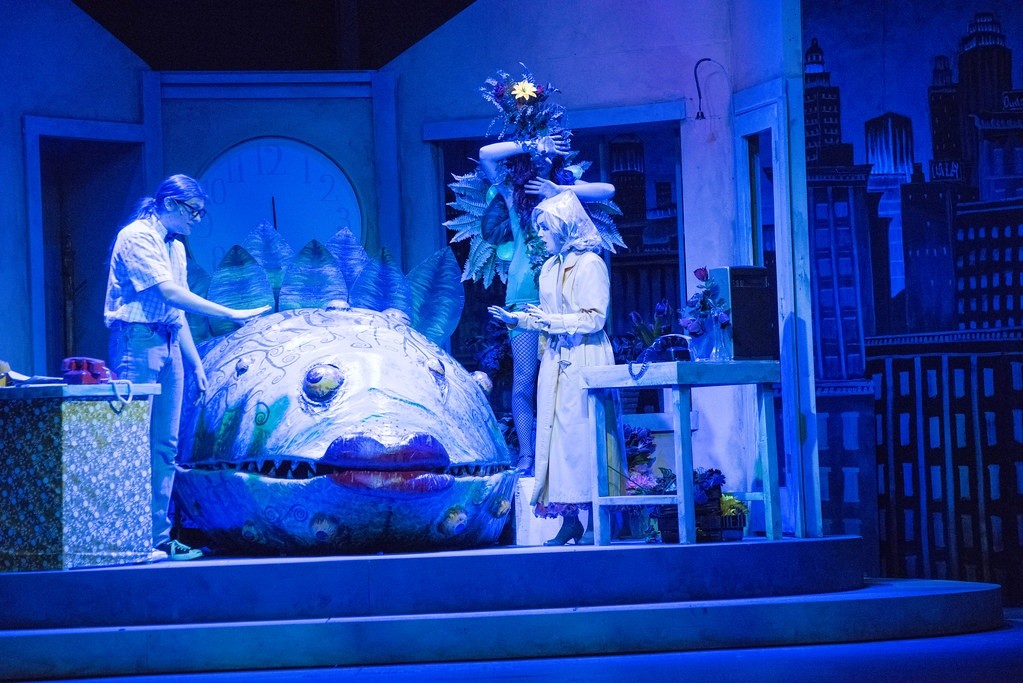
[622,500,658,539]
[722,512,745,541]
[658,502,721,540]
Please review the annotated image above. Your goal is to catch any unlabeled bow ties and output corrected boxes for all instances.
[164,232,178,243]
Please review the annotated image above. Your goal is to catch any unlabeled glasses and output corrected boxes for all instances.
[163,196,207,218]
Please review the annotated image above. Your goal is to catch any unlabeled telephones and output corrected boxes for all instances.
[635,333,700,363]
[61,356,118,387]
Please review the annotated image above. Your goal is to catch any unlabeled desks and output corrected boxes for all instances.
[581,357,781,544]
[0,382,161,570]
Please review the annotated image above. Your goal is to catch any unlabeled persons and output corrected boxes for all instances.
[487,189,627,546]
[103,175,272,563]
[479,133,616,476]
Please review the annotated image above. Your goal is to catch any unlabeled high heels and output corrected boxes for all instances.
[578,525,594,545]
[544,521,584,546]
[515,454,534,477]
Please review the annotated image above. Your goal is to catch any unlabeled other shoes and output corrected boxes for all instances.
[471,370,493,396]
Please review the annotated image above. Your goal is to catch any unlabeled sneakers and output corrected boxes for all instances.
[153,539,203,562]
[152,546,168,559]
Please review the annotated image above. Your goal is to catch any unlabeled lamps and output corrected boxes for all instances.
[693,58,732,121]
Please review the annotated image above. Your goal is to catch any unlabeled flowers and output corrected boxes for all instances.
[482,66,573,139]
[621,468,747,515]
